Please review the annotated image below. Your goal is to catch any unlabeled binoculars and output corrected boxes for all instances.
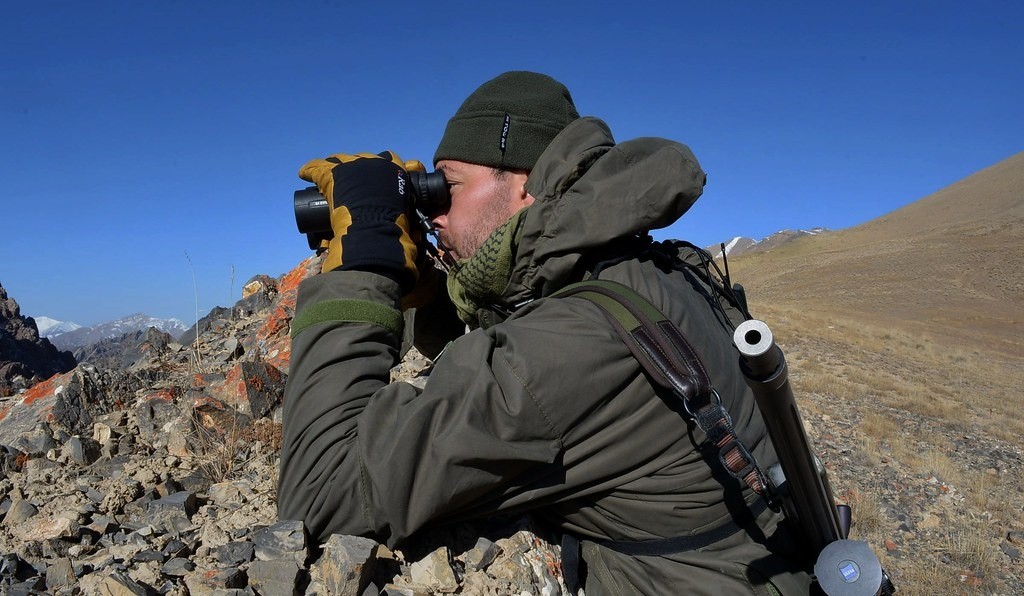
[293,171,449,251]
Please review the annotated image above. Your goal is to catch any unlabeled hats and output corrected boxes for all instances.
[432,70,580,171]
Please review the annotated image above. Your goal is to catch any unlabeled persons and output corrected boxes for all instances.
[275,71,816,596]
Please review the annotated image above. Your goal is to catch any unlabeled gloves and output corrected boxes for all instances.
[298,150,450,313]
